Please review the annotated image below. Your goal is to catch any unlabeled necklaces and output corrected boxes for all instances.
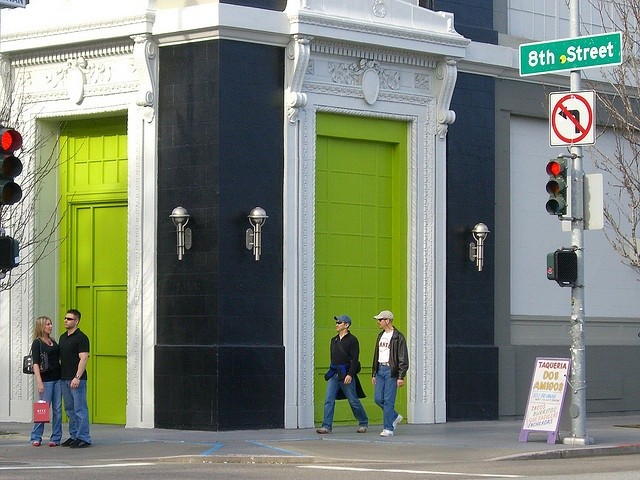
[42,337,51,345]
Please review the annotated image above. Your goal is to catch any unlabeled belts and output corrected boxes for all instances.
[378,363,391,366]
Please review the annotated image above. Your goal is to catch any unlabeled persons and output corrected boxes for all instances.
[371,310,409,437]
[58,310,91,447]
[316,315,369,434]
[30,316,63,446]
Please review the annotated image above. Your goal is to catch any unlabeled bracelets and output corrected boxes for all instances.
[76,374,82,380]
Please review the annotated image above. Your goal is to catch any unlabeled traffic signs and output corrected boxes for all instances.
[519,31,622,77]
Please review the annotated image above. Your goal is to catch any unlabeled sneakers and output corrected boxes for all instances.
[316,427,333,434]
[392,414,404,431]
[380,429,394,436]
[61,438,75,447]
[357,427,366,433]
[70,438,91,448]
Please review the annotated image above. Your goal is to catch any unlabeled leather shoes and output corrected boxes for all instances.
[48,442,56,447]
[32,441,41,447]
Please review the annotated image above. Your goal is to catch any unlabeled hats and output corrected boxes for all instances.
[374,310,393,320]
[334,314,352,324]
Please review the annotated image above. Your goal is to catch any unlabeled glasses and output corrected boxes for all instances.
[336,322,344,324]
[378,319,387,322]
[65,317,76,320]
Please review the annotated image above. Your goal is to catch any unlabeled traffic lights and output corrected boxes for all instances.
[546,159,567,215]
[0,236,19,269]
[0,125,22,205]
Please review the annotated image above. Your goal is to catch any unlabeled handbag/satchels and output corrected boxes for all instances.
[32,392,50,423]
[23,339,52,374]
